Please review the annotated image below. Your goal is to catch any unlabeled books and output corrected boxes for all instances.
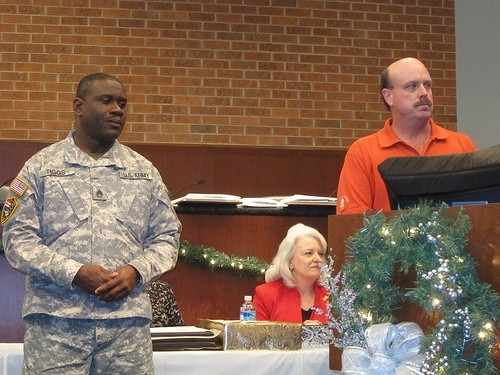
[145,321,223,349]
[171,192,339,208]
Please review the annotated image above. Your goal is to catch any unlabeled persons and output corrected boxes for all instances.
[252,223,331,325]
[0,71,184,375]
[335,58,482,215]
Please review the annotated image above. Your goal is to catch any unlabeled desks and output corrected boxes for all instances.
[0,335,332,375]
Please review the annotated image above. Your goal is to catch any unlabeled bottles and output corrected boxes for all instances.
[240,296,256,321]
